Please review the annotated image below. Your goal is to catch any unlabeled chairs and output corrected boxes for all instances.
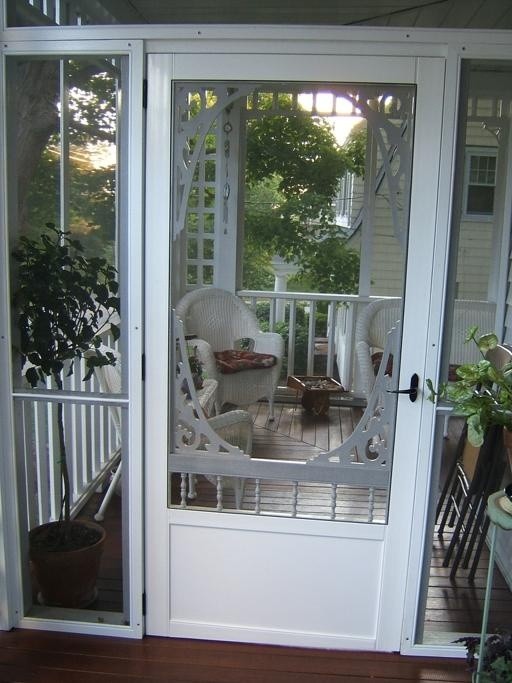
[86,343,255,522]
[433,344,512,579]
[174,288,285,422]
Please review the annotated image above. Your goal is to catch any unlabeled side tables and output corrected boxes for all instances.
[184,379,219,419]
[475,489,511,682]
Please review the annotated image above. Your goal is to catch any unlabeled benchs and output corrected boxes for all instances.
[355,300,512,445]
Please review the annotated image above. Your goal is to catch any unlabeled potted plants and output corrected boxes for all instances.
[10,222,120,607]
[176,356,204,388]
[426,326,512,474]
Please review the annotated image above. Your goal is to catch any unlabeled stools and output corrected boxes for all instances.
[287,375,344,415]
